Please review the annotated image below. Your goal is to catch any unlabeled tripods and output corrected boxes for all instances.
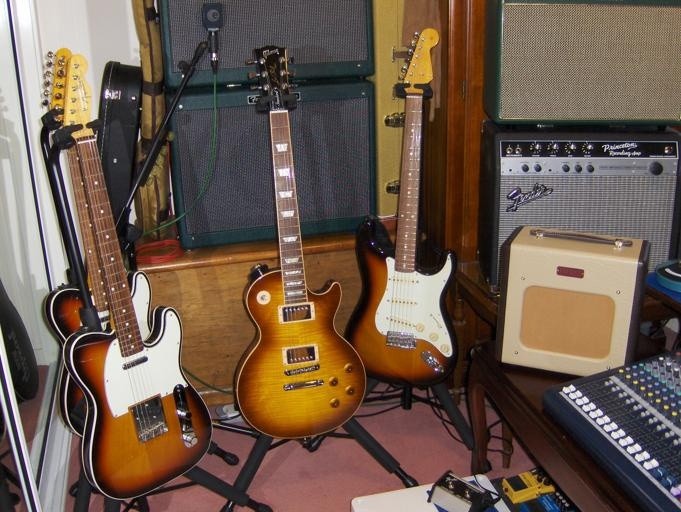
[306,86,491,476]
[219,415,418,512]
[38,108,273,512]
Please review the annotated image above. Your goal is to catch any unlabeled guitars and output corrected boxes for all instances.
[40,48,151,436]
[234,43,365,439]
[345,27,455,390]
[55,54,213,499]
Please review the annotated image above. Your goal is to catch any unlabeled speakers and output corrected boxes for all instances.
[480,122,681,295]
[482,0,681,130]
[163,78,376,252]
[157,0,375,90]
[496,226,650,379]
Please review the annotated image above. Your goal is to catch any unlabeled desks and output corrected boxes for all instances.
[466,271,681,512]
[453,270,676,471]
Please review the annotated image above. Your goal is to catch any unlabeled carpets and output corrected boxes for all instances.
[0,367,535,512]
